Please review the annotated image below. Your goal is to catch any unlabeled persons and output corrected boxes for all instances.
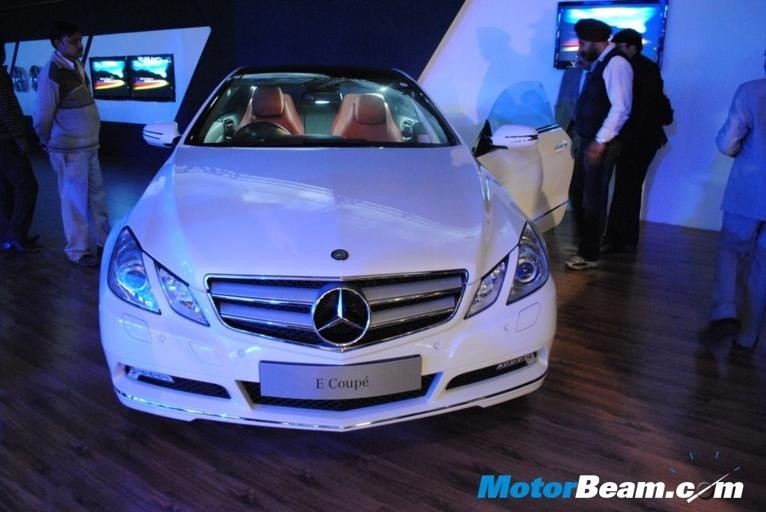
[33,24,111,268]
[599,29,674,256]
[564,19,634,270]
[0,44,40,254]
[707,49,766,351]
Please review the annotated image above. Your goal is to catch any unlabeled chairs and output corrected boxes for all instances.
[330,92,402,142]
[236,86,304,136]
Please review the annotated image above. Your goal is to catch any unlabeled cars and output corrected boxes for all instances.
[99,67,575,434]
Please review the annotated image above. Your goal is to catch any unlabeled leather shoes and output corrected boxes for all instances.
[2,235,40,254]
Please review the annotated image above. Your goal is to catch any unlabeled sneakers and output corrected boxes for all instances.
[69,246,104,266]
[563,242,599,270]
[698,318,754,355]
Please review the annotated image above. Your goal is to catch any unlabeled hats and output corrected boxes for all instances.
[574,19,611,42]
[610,28,642,43]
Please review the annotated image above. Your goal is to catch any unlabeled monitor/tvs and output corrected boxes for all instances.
[89,53,176,102]
[554,0,670,71]
